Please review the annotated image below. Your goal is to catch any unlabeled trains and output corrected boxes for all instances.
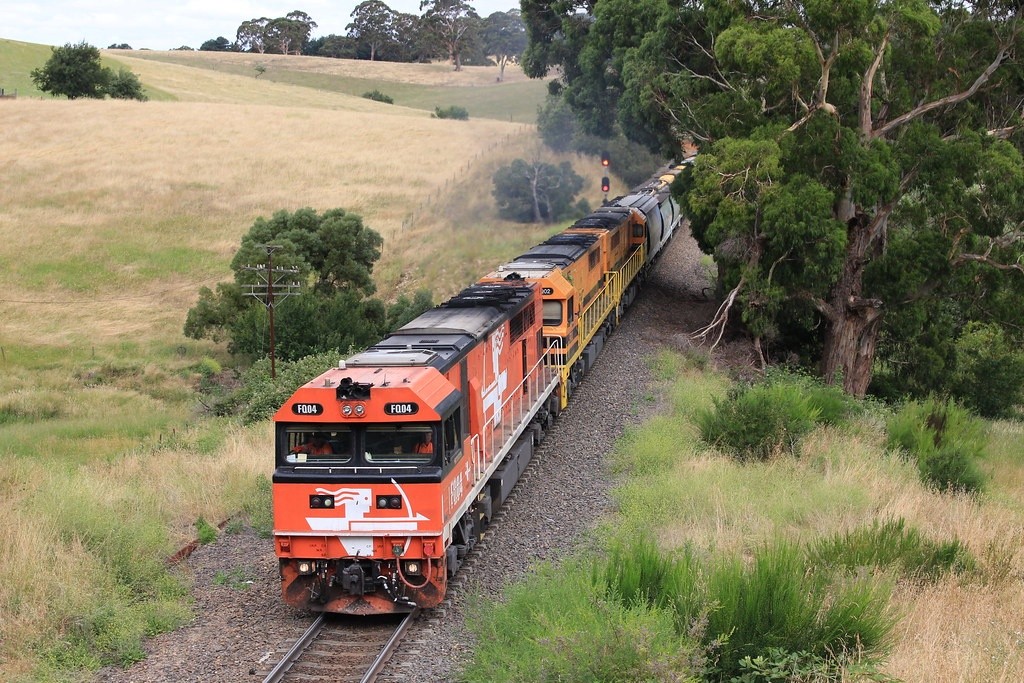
[272,152,698,614]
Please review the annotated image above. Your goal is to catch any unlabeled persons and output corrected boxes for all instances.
[416,433,433,453]
[290,433,332,454]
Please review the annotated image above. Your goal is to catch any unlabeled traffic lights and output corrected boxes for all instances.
[602,150,610,166]
[602,177,609,192]
